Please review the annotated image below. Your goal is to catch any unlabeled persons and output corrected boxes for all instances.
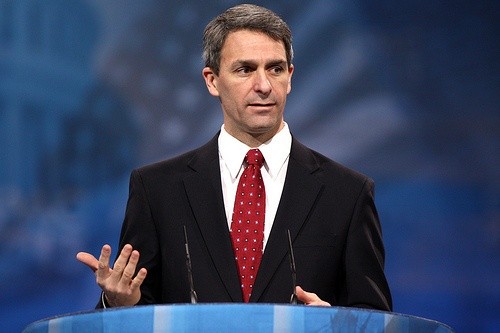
[76,5,394,310]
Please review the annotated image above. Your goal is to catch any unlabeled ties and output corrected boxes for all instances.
[229,149,267,303]
